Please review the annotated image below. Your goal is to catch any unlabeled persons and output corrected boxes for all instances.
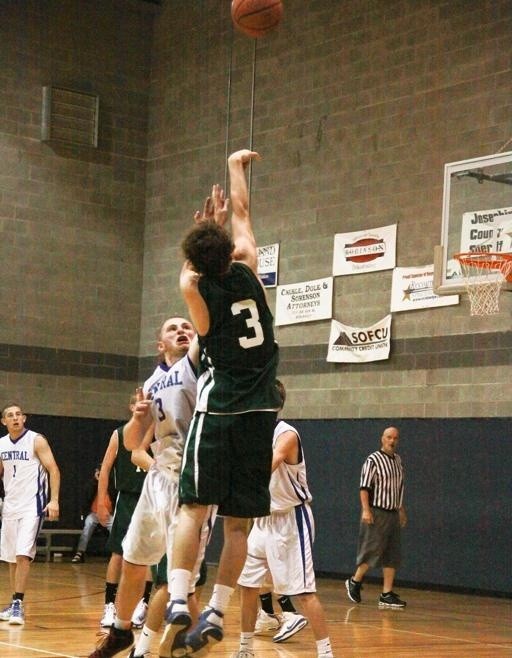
[88,184,231,657]
[253,569,309,646]
[71,463,116,564]
[234,378,335,658]
[127,543,209,658]
[344,427,410,608]
[97,386,156,631]
[0,400,63,625]
[159,149,278,657]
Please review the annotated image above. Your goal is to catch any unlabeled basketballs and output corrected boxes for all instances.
[231,0,283,36]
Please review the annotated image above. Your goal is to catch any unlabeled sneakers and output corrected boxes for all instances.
[101,602,115,626]
[188,606,225,658]
[8,599,24,625]
[378,592,406,607]
[132,598,148,628]
[272,612,308,642]
[72,551,84,563]
[255,608,281,633]
[0,603,12,620]
[345,576,361,603]
[88,623,135,657]
[159,599,192,657]
